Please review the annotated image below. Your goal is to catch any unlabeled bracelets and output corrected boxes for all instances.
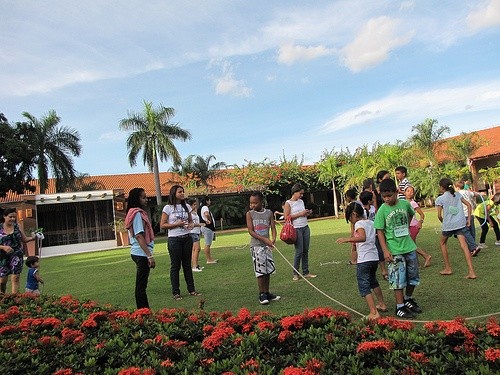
[147,255,154,259]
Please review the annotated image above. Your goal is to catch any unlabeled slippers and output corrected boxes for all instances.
[361,315,383,322]
[471,246,481,257]
[346,260,358,265]
[375,304,388,313]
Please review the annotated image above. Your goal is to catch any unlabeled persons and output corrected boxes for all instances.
[344,166,431,280]
[434,178,477,280]
[186,199,205,273]
[125,187,156,311]
[452,178,500,257]
[283,184,319,281]
[375,177,423,318]
[200,196,218,264]
[24,256,44,297]
[160,185,201,300]
[336,202,387,323]
[0,208,44,294]
[246,191,281,304]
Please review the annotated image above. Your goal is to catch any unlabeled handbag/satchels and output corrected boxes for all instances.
[213,233,216,241]
[280,215,296,245]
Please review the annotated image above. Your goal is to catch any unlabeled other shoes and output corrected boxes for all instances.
[293,274,299,281]
[304,273,318,278]
[207,259,219,264]
[258,293,269,304]
[495,241,500,246]
[192,265,205,271]
[478,242,489,249]
[189,291,202,297]
[382,273,389,281]
[173,294,182,301]
[268,293,281,301]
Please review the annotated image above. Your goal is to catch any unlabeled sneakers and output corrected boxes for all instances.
[403,297,422,314]
[395,304,415,318]
